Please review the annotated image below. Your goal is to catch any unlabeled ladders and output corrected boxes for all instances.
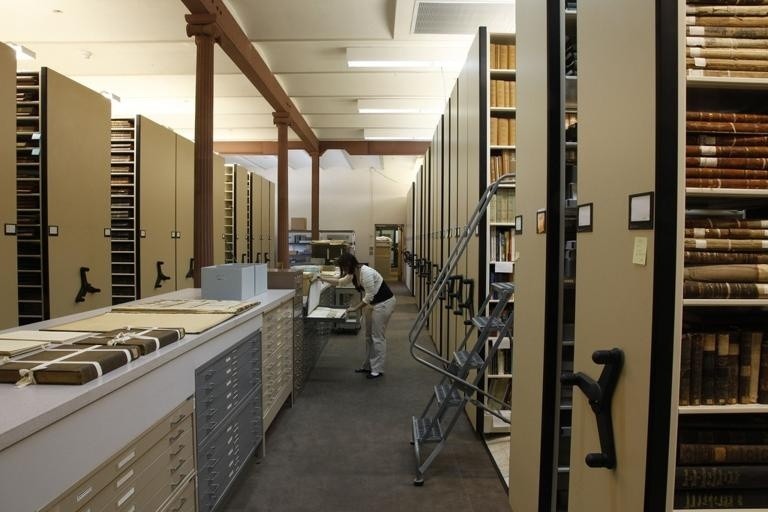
[406,174,517,486]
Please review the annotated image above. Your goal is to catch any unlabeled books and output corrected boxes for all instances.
[556,1,579,470]
[0,339,50,358]
[16,73,39,238]
[291,263,346,278]
[0,344,140,386]
[72,326,185,356]
[486,38,514,413]
[110,119,134,240]
[0,330,90,345]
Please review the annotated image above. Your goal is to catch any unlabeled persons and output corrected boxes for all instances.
[316,252,397,380]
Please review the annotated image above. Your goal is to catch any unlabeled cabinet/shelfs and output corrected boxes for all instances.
[569,0,768,510]
[441,82,454,369]
[405,166,423,310]
[17,66,113,323]
[268,269,335,402]
[422,116,441,353]
[507,2,577,510]
[279,230,355,266]
[213,152,227,266]
[247,172,276,268]
[39,394,197,511]
[194,326,263,511]
[456,26,511,438]
[0,42,19,330]
[225,163,249,264]
[263,298,295,441]
[110,114,176,300]
[175,135,196,291]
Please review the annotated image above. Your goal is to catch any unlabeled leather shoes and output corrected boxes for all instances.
[355,368,369,373]
[366,371,382,379]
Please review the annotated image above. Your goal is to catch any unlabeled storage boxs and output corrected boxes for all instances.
[201,265,255,300]
[232,262,268,295]
